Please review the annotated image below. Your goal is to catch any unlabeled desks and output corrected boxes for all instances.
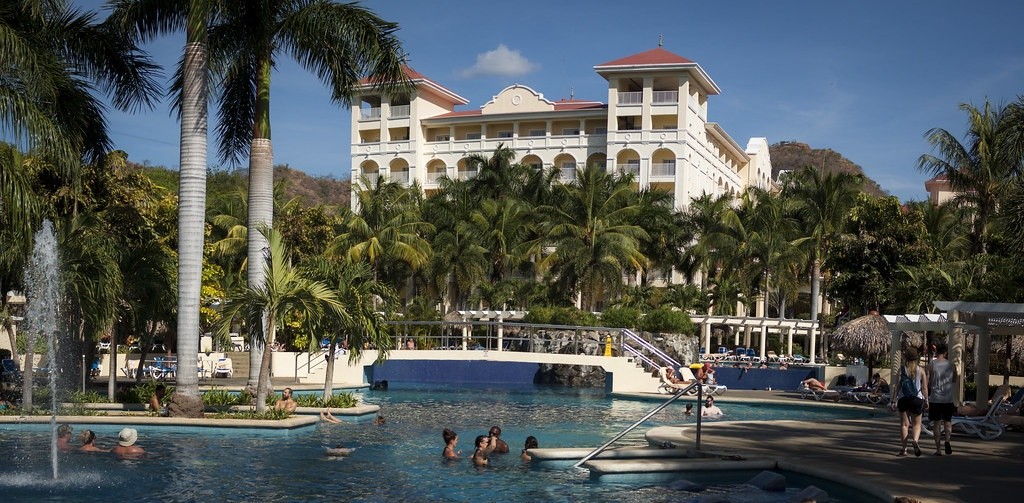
[144,360,178,363]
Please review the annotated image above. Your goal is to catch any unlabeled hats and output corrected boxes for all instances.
[118,428,138,447]
[905,348,918,361]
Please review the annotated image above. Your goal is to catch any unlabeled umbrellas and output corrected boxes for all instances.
[830,311,923,382]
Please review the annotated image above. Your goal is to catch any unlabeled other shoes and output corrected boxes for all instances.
[934,452,942,456]
[899,450,908,456]
[945,442,952,455]
[912,441,921,456]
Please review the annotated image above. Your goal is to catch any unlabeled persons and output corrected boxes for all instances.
[891,343,956,457]
[666,368,691,384]
[473,427,509,464]
[80,430,109,452]
[275,388,296,413]
[685,403,694,415]
[150,385,165,410]
[320,410,384,424]
[733,360,788,370]
[871,374,890,393]
[58,424,72,449]
[953,403,992,418]
[111,428,144,453]
[803,378,827,391]
[443,429,462,457]
[521,436,538,460]
[407,338,414,349]
[696,366,717,385]
[701,396,722,417]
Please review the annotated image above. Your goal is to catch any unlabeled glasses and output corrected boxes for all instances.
[489,431,498,436]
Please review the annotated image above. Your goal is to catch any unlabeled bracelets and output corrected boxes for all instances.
[891,402,895,403]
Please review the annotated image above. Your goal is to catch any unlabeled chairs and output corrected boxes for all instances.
[918,384,1010,439]
[121,359,149,378]
[801,385,892,405]
[658,366,727,396]
[700,348,808,362]
[152,356,176,379]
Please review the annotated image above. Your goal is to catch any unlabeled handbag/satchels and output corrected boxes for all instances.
[901,365,922,400]
